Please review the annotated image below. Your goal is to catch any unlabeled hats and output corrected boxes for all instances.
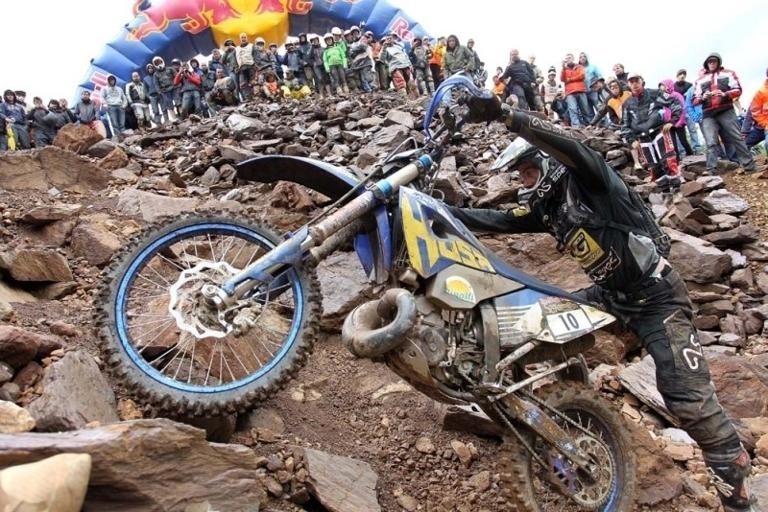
[626,71,641,81]
[547,68,555,75]
[589,75,604,88]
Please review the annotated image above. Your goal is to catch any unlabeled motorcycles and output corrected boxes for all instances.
[92,70,637,512]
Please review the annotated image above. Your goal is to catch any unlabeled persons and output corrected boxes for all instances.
[0,23,768,196]
[432,88,758,511]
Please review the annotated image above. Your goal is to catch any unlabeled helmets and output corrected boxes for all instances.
[254,25,374,51]
[490,137,568,208]
[151,55,165,71]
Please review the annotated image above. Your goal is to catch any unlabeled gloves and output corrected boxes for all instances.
[459,89,501,126]
